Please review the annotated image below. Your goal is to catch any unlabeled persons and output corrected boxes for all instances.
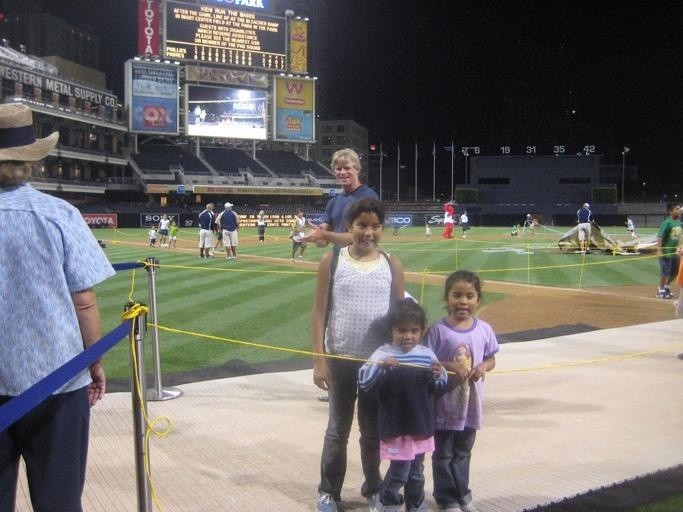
[290,208,307,262]
[424,270,499,512]
[526,214,536,234]
[425,215,433,239]
[200,203,215,259]
[188,102,232,131]
[256,211,266,242]
[673,207,683,313]
[511,224,520,238]
[441,212,453,240]
[149,224,157,247]
[625,216,637,237]
[0,102,116,512]
[312,197,404,511]
[460,211,468,238]
[214,230,222,251]
[299,149,381,403]
[443,200,459,215]
[657,201,683,299]
[168,221,179,250]
[158,214,171,248]
[215,203,240,260]
[575,202,594,255]
[358,298,448,511]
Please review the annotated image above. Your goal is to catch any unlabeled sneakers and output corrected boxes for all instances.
[442,504,461,512]
[367,495,377,512]
[656,290,670,298]
[461,501,479,512]
[318,492,338,512]
[665,288,673,296]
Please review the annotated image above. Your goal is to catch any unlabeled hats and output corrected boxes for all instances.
[0,103,59,162]
[224,203,233,207]
